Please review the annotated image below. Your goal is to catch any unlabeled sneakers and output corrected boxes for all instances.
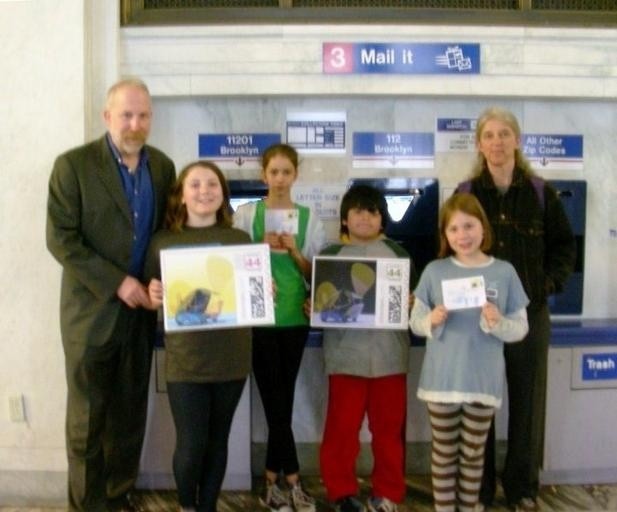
[288,479,316,512]
[334,495,397,512]
[259,476,293,512]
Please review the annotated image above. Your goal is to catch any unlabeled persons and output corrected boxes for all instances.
[143,161,254,512]
[440,106,579,511]
[234,141,325,511]
[44,77,178,512]
[409,189,532,511]
[303,180,420,511]
